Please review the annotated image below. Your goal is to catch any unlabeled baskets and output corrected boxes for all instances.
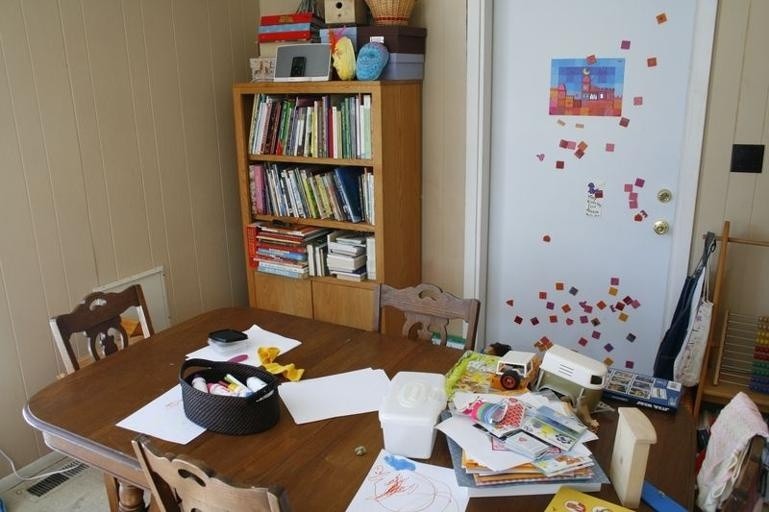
[179,358,279,435]
[365,0,417,25]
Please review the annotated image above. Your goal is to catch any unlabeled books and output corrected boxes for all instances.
[243,93,376,282]
[442,349,503,401]
[543,484,637,512]
[603,366,684,415]
[433,388,612,497]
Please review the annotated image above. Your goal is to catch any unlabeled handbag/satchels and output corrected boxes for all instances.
[653,232,715,388]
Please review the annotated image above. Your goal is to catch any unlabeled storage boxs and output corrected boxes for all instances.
[377,369,448,462]
[245,0,428,81]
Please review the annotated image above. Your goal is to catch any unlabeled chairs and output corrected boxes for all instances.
[695,391,769,512]
[47,283,157,512]
[366,281,480,353]
[128,431,292,512]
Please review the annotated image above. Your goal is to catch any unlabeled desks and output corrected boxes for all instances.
[20,305,699,512]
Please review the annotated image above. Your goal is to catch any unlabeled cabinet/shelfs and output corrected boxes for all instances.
[230,75,425,342]
